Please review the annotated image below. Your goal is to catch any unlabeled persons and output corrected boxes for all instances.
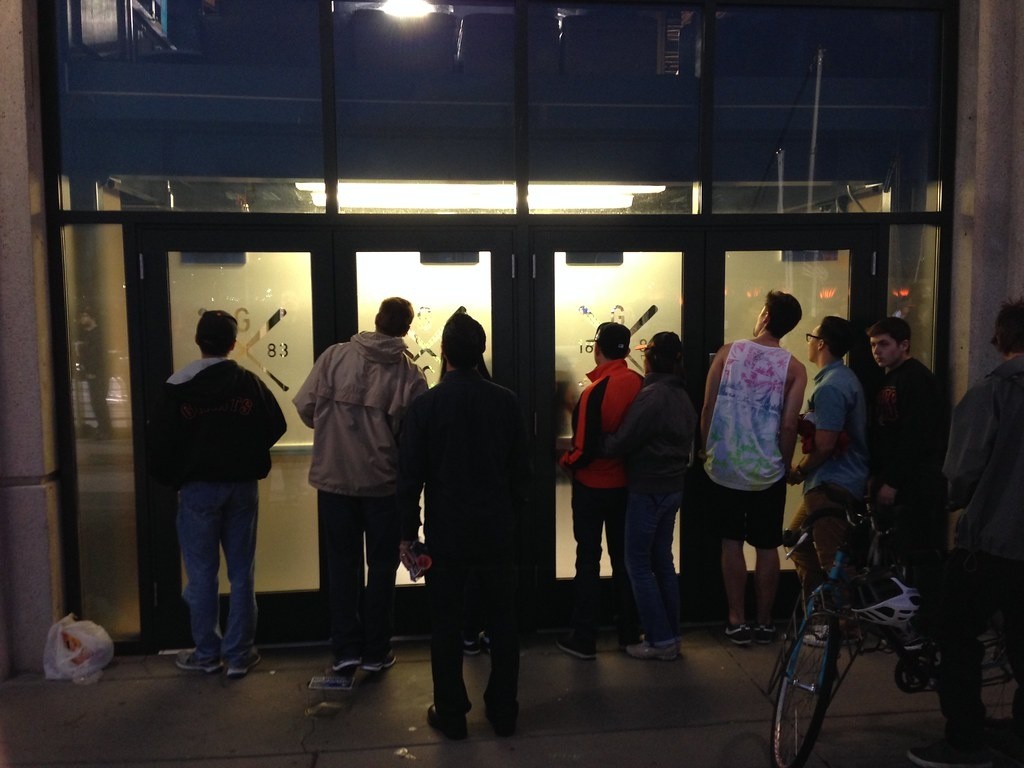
[905,297,1024,768]
[599,331,698,660]
[553,370,581,433]
[698,292,807,646]
[784,317,866,649]
[868,318,944,652]
[553,323,645,657]
[291,298,429,673]
[397,312,537,741]
[76,304,117,441]
[149,310,288,677]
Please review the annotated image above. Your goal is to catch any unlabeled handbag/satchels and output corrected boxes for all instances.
[42,612,114,681]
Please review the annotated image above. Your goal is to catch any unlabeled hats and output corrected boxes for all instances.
[635,331,681,358]
[585,322,630,350]
[196,308,238,350]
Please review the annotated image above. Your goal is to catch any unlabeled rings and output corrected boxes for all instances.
[402,554,406,556]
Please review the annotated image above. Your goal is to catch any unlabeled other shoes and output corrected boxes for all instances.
[485,699,515,739]
[427,702,467,740]
[465,634,490,656]
[555,635,595,660]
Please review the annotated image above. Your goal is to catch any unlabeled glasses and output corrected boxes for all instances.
[805,332,829,346]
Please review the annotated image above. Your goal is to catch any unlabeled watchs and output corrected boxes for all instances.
[797,465,805,477]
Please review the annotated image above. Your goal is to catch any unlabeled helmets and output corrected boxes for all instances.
[840,571,920,624]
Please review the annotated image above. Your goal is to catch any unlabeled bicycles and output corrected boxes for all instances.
[768,471,1016,768]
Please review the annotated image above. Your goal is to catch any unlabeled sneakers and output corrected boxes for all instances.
[722,620,752,645]
[908,740,987,767]
[756,623,778,644]
[802,625,830,647]
[840,626,864,644]
[228,648,260,680]
[628,634,679,659]
[178,645,221,673]
[361,652,395,671]
[990,734,1024,766]
[331,654,359,672]
